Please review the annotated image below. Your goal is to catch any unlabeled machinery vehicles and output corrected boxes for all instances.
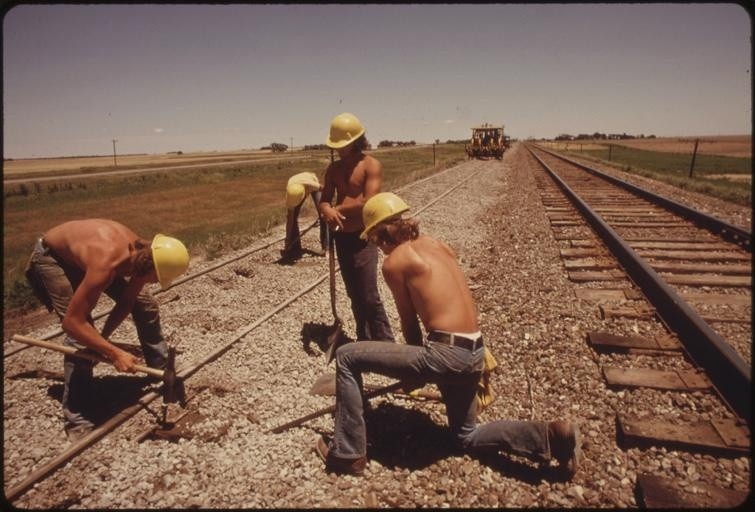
[465,123,510,160]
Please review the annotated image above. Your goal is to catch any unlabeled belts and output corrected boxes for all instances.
[427,331,483,351]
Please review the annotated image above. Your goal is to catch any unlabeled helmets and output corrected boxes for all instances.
[326,113,366,149]
[285,182,305,209]
[359,192,411,240]
[150,233,190,291]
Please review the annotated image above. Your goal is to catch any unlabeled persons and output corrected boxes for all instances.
[281,173,327,258]
[25,215,190,425]
[317,112,394,343]
[317,191,578,479]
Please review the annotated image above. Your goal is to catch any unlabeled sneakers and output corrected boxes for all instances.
[548,421,581,481]
[316,435,364,476]
[63,405,96,431]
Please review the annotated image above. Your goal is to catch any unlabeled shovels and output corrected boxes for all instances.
[326,222,343,365]
[309,374,445,402]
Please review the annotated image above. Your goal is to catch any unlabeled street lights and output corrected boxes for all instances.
[111,138,119,168]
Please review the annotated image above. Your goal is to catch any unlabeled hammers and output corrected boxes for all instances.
[13,334,176,404]
